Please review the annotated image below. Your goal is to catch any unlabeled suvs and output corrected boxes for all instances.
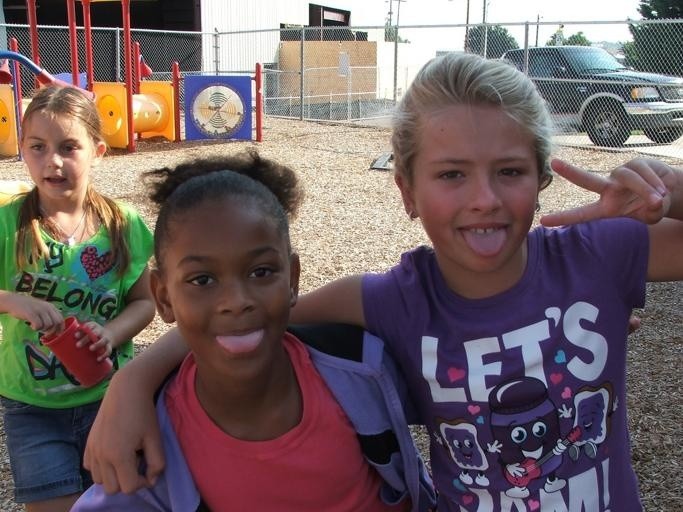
[496,46,683,145]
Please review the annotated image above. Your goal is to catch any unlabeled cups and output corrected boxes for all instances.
[37,316,112,390]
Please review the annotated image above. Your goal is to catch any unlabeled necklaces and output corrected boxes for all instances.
[39,206,93,246]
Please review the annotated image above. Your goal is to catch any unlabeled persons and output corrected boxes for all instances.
[81,50,683,512]
[2,84,159,510]
[71,147,641,510]
